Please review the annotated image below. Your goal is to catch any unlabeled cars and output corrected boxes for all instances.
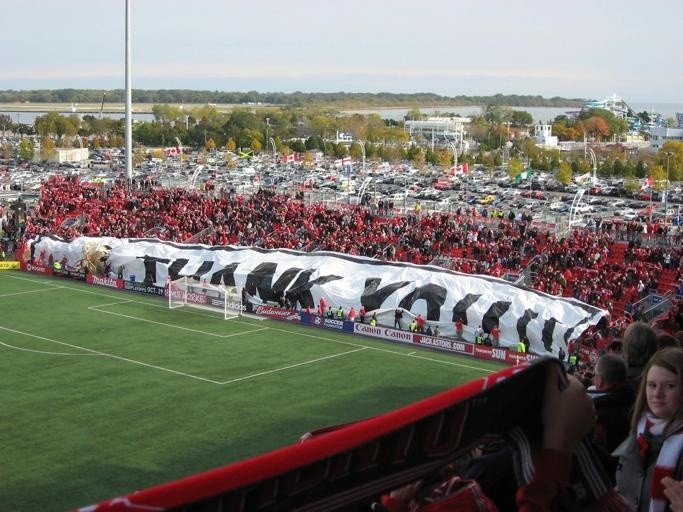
[264,156,549,221]
[90,147,125,174]
[550,171,683,230]
[161,147,260,193]
[0,163,80,191]
